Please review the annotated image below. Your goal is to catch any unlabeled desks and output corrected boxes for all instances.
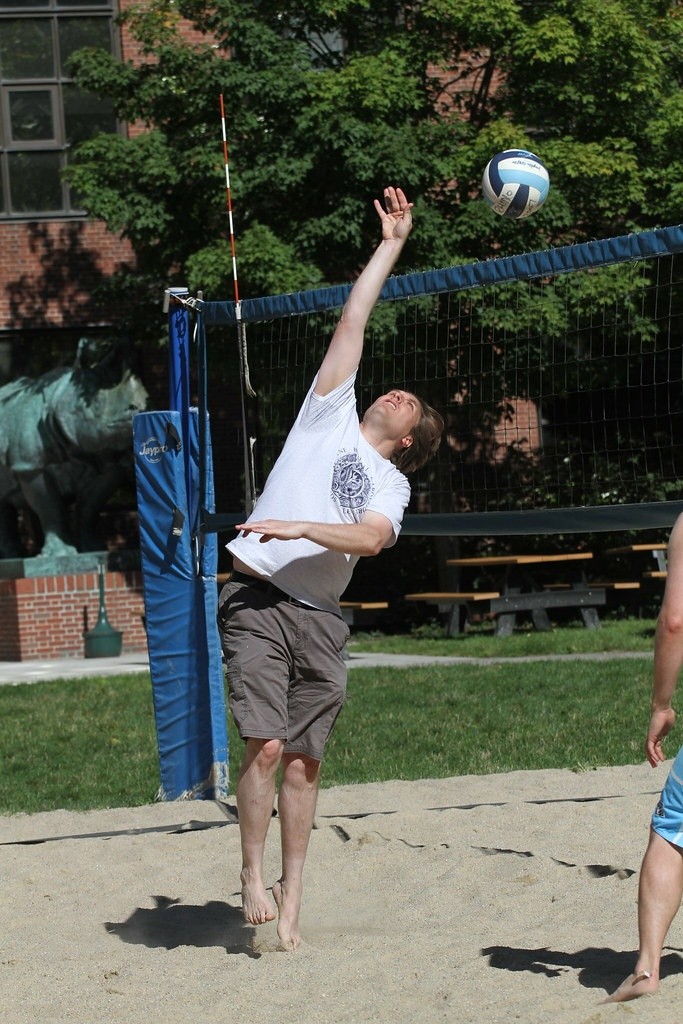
[445,553,600,638]
[605,542,669,620]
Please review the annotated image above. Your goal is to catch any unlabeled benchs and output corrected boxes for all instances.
[644,570,671,580]
[405,591,502,604]
[339,599,389,610]
[543,581,640,591]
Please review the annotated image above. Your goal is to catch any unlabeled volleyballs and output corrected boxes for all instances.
[481,145,553,223]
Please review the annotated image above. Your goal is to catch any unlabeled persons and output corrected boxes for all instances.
[218,186,444,949]
[600,511,683,1003]
